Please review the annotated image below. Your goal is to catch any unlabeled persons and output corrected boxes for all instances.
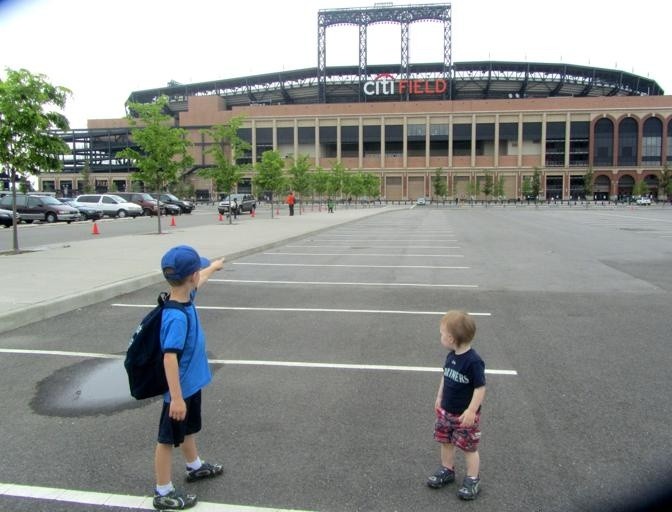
[426,307,487,502]
[286,193,295,216]
[227,198,239,219]
[327,196,334,213]
[153,245,225,512]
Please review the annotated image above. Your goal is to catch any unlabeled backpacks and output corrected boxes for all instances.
[124,292,193,400]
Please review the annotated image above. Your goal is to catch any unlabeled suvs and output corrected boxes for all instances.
[217,193,258,215]
[635,196,652,205]
[0,187,195,227]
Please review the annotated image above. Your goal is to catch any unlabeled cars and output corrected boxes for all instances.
[416,197,427,206]
[620,194,634,202]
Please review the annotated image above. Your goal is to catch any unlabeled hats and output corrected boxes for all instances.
[162,245,210,279]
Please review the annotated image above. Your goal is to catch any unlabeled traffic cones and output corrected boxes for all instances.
[250,207,256,217]
[274,205,327,214]
[90,222,101,235]
[216,213,224,221]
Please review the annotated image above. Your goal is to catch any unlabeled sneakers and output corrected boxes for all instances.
[153,484,198,510]
[426,466,455,488]
[184,460,224,483]
[457,473,481,501]
